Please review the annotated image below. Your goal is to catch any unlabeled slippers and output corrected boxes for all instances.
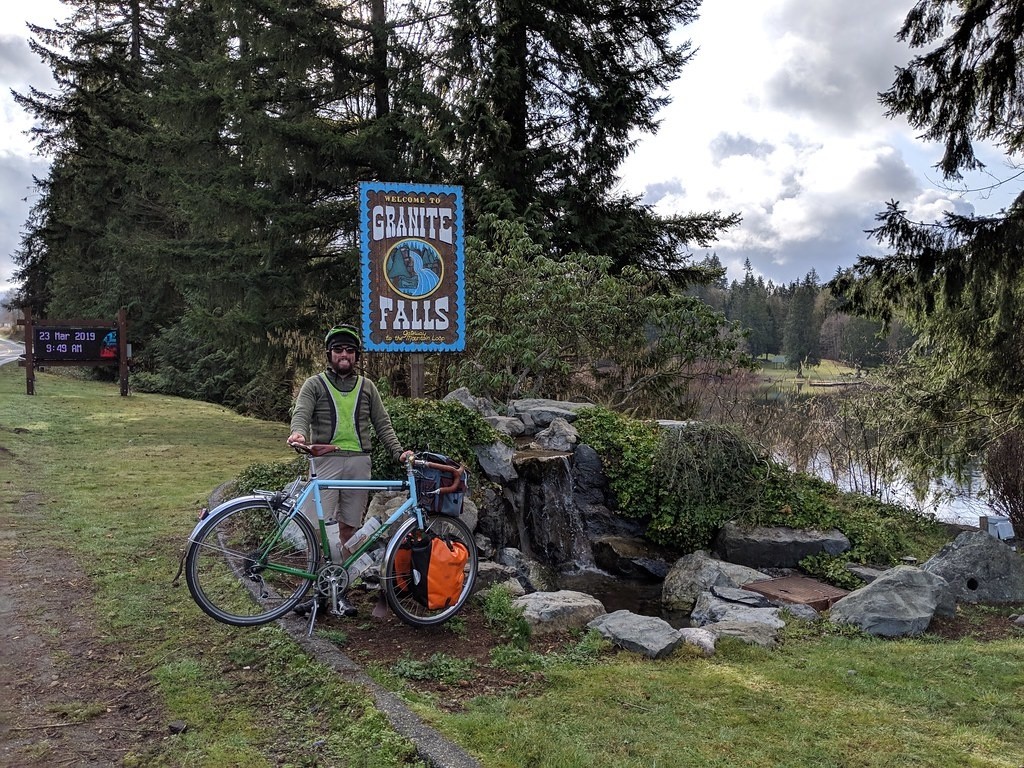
[325,517,337,525]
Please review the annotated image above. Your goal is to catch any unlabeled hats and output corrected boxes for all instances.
[327,334,358,350]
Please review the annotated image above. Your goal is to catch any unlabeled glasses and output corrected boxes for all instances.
[333,324,359,332]
[330,346,357,354]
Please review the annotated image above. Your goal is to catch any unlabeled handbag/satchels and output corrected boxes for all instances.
[411,533,470,610]
[394,526,437,598]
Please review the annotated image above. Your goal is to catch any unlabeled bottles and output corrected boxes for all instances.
[344,515,383,552]
[325,518,345,564]
[339,552,376,589]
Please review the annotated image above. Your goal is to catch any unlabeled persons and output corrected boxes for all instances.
[287,324,415,619]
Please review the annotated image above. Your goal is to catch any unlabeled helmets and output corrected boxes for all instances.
[325,327,361,353]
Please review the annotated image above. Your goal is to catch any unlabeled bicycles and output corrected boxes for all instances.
[168,441,479,639]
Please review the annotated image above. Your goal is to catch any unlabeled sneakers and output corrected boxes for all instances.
[293,591,329,616]
[337,595,360,618]
